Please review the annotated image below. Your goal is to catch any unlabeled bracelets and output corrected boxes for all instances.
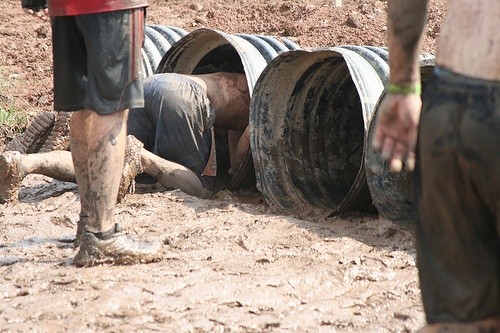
[387,83,421,96]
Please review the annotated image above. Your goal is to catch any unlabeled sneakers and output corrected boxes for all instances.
[116,134,145,203]
[37,110,74,150]
[0,151,23,204]
[75,224,162,266]
[6,112,55,154]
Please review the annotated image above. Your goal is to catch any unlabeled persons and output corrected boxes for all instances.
[18,0,168,268]
[369,0,499,332]
[0,72,253,207]
